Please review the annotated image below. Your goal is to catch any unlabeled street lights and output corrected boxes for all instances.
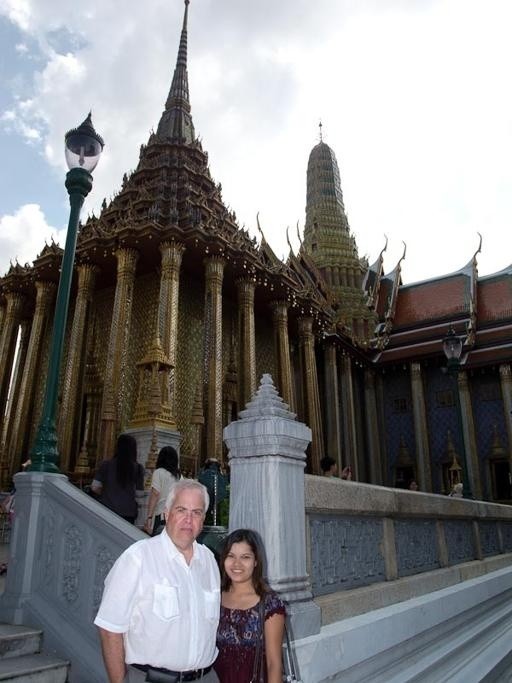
[443,323,475,498]
[25,110,105,474]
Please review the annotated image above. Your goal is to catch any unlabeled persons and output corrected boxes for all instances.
[448,483,463,498]
[145,446,181,537]
[212,528,286,683]
[93,479,221,682]
[198,460,226,526]
[320,456,352,481]
[91,435,145,525]
[408,479,418,491]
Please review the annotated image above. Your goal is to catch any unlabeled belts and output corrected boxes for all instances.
[130,661,215,681]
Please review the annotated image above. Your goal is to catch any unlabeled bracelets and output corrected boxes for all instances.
[148,516,152,519]
